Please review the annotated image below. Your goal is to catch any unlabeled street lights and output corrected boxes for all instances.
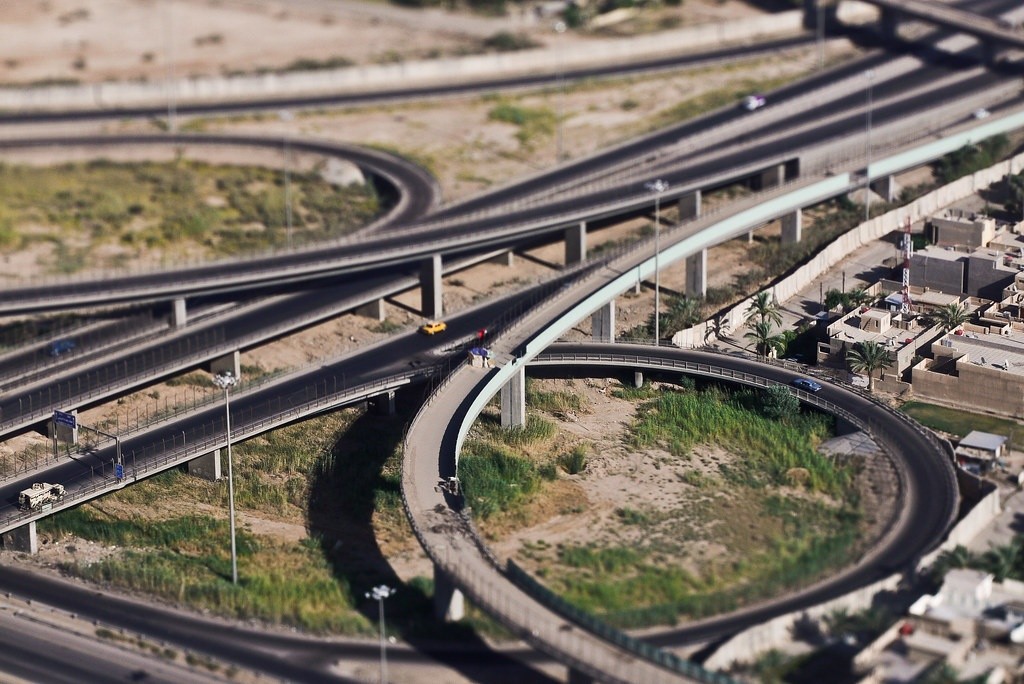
[643,176,670,350]
[365,583,396,684]
[212,370,238,585]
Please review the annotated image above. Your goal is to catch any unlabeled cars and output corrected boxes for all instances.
[421,321,447,336]
[792,378,821,393]
[46,342,75,356]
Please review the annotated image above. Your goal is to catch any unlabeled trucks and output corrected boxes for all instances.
[18,482,64,510]
